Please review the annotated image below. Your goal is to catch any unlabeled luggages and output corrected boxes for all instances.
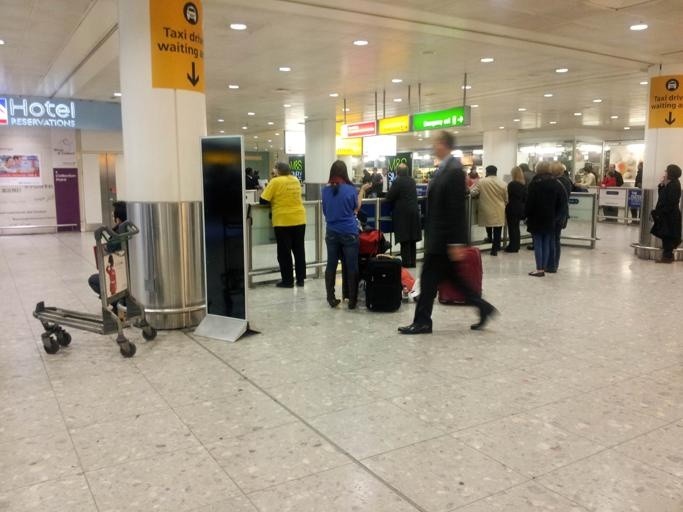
[438,241,483,304]
[366,253,402,311]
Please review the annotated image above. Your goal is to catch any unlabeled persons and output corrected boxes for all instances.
[630,162,642,224]
[575,167,596,189]
[259,162,306,287]
[105,256,117,295]
[504,166,527,252]
[519,163,534,185]
[602,163,624,223]
[469,165,508,256]
[397,130,502,334]
[361,169,371,184]
[246,168,260,190]
[384,163,422,268]
[649,165,682,264]
[371,168,383,184]
[467,165,480,180]
[87,201,129,300]
[521,160,572,277]
[320,160,373,309]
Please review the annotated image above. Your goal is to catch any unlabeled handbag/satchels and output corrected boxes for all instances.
[359,230,382,256]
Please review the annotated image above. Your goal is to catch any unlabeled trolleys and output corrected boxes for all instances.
[32,220,157,358]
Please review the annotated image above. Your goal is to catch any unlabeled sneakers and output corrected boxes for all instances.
[655,257,671,264]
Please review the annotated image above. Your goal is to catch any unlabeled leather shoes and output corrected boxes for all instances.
[470,302,495,329]
[529,272,544,277]
[347,300,357,308]
[330,298,340,307]
[398,319,432,333]
[297,278,304,286]
[527,245,534,249]
[277,279,293,288]
